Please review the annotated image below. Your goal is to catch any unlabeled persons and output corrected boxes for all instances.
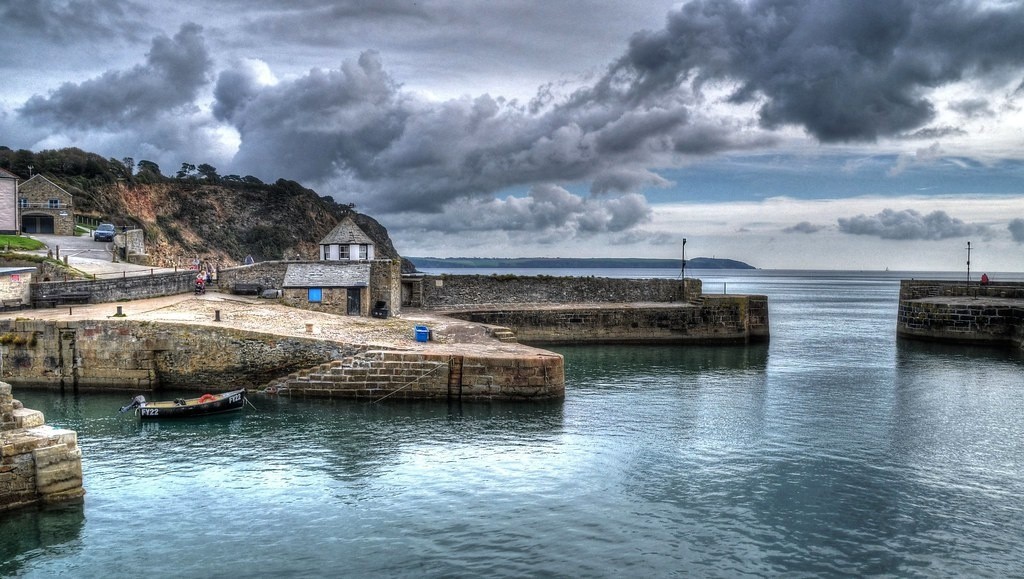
[981,273,989,286]
[245,254,254,264]
[196,257,213,289]
[122,223,129,232]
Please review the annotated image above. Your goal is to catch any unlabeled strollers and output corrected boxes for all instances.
[195,279,206,295]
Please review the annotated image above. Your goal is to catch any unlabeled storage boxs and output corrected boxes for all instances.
[415,326,429,342]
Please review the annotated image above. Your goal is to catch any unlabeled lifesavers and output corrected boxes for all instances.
[198,394,216,403]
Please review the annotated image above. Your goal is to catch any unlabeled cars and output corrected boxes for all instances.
[94,223,116,242]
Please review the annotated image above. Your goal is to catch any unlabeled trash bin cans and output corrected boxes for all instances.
[415,326,430,342]
[119,246,125,259]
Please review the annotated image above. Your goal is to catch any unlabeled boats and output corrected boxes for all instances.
[119,388,246,422]
